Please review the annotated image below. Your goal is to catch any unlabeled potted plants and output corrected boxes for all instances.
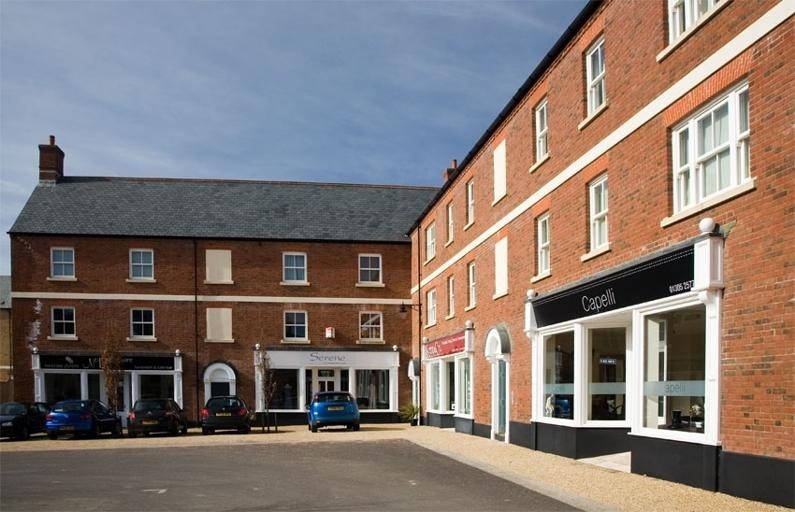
[399,403,419,426]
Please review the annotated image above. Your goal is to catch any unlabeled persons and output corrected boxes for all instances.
[545,394,555,418]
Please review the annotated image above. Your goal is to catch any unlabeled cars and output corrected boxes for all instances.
[127,398,187,438]
[306,391,360,432]
[202,395,251,435]
[0,399,122,440]
